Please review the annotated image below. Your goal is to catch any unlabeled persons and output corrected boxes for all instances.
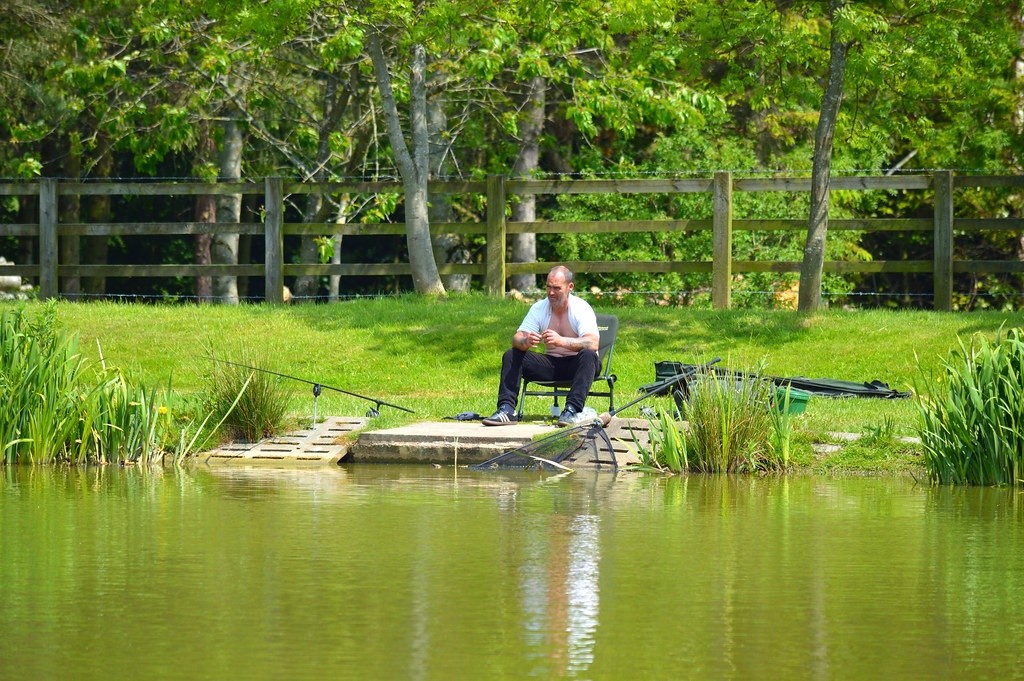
[481,266,600,427]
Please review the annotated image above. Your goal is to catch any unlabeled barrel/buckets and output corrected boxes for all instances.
[779,385,810,416]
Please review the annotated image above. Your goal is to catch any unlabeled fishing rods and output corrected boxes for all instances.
[195,354,416,418]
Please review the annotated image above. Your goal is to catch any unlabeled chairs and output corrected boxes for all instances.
[518,314,618,420]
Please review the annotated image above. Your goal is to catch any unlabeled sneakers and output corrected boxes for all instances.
[558,406,577,427]
[481,407,518,425]
